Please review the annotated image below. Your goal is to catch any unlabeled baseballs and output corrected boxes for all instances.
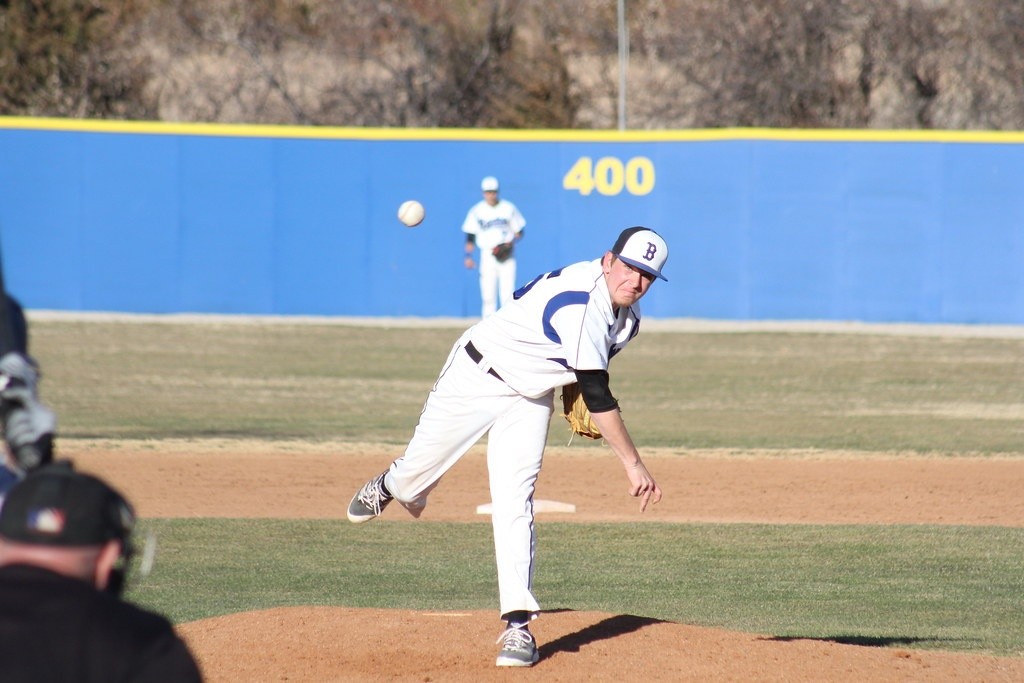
[397,199,426,228]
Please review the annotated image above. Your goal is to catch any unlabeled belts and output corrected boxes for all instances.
[464,340,502,382]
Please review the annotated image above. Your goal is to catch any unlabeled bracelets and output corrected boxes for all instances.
[465,252,473,257]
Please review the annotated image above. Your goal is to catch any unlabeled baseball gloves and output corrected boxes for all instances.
[492,242,514,262]
[562,386,620,440]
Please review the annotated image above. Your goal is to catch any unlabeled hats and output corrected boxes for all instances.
[611,226,670,282]
[481,177,499,192]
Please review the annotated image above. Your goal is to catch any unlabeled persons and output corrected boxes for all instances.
[0,460,201,683]
[462,176,526,322]
[346,226,669,667]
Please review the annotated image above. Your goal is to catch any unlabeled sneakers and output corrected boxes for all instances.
[346,468,394,524]
[494,619,540,667]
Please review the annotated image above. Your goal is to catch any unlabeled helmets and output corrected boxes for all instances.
[0,473,137,545]
